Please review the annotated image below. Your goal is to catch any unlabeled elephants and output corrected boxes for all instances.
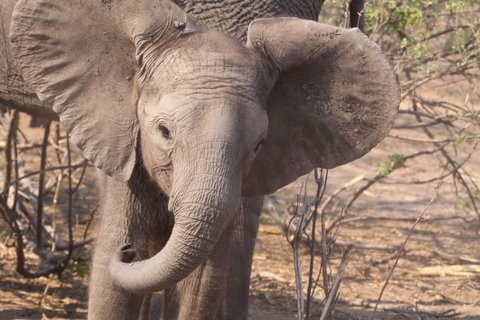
[0,0,400,320]
